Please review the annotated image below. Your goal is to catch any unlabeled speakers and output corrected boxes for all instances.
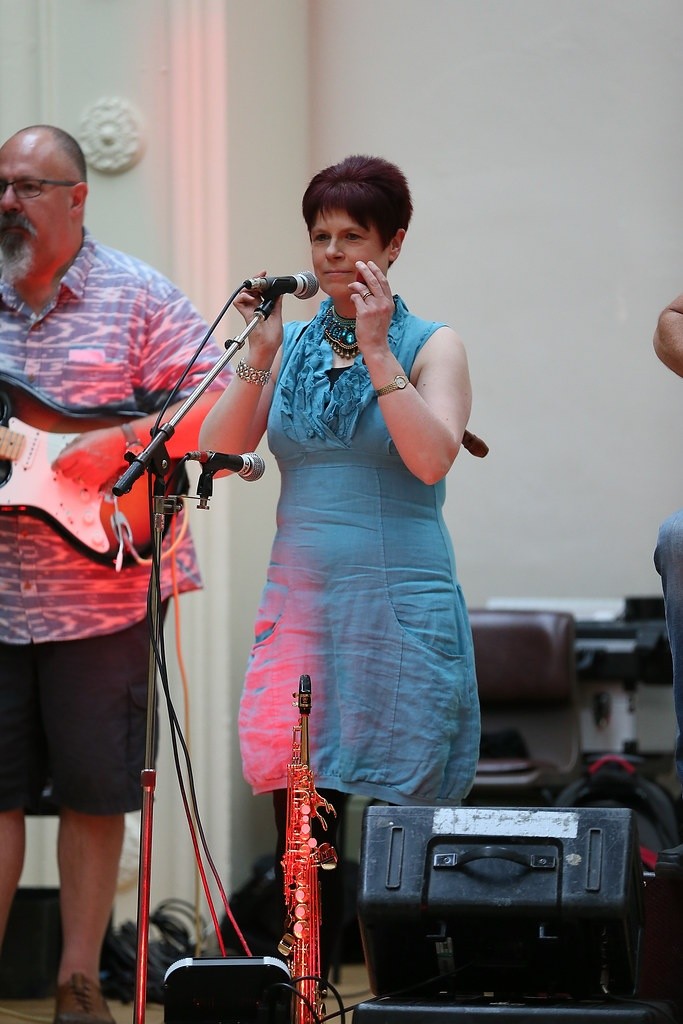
[0,886,73,1001]
[358,804,647,1001]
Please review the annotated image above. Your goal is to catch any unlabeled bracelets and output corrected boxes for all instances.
[235,356,273,386]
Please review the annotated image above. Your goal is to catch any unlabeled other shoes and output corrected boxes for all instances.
[655,844,683,879]
[55,973,116,1024]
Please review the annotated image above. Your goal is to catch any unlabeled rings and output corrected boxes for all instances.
[362,291,373,300]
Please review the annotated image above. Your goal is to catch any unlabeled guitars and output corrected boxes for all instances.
[1,372,191,569]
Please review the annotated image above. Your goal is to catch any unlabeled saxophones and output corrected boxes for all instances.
[278,673,340,1024]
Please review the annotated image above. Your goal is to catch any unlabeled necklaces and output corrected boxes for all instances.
[322,304,361,360]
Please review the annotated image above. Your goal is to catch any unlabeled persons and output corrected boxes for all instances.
[199,158,472,999]
[653,292,683,876]
[0,125,233,1024]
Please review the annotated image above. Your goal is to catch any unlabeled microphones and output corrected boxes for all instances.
[184,448,266,482]
[242,271,320,300]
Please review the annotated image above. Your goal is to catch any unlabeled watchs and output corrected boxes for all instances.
[376,374,410,397]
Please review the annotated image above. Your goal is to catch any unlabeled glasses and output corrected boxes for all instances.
[0,179,76,199]
